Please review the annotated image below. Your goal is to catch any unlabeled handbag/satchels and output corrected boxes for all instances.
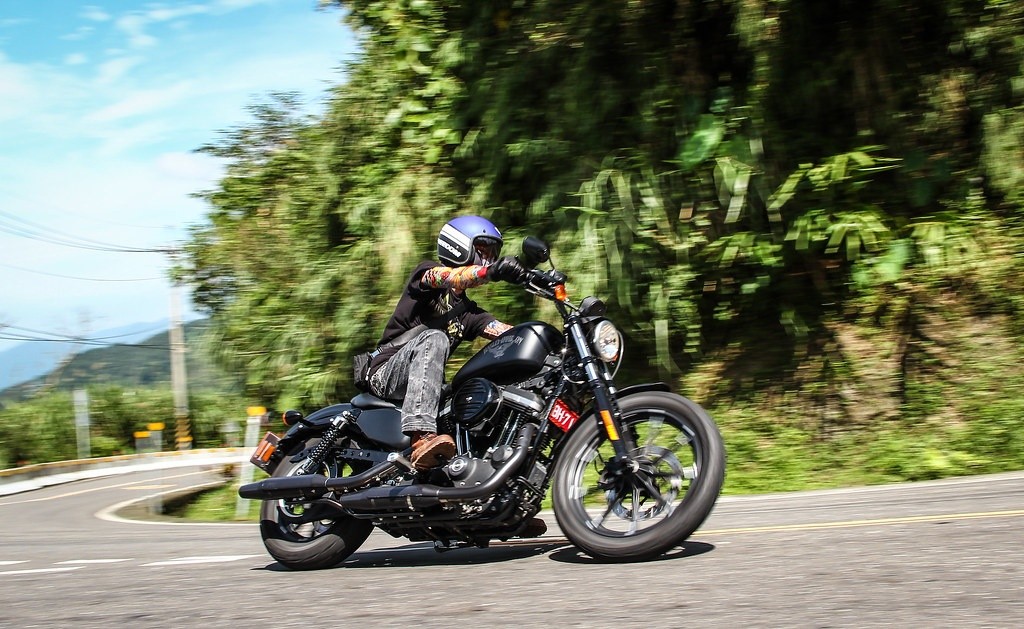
[351,351,376,392]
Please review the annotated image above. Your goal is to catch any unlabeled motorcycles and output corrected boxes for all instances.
[239,243,727,569]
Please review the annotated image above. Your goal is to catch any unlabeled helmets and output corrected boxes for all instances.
[438,215,504,267]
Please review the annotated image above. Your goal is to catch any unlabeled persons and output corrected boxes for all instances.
[368,216,548,541]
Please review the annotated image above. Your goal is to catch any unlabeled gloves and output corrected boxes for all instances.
[486,255,528,286]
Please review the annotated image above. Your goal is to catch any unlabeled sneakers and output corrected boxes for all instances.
[410,429,458,472]
